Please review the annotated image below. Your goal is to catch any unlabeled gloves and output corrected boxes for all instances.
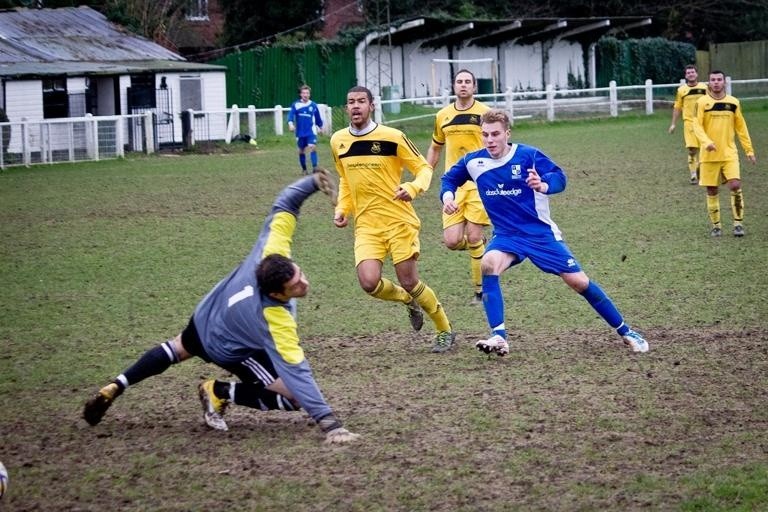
[314,167,337,206]
[323,428,361,447]
[668,125,675,134]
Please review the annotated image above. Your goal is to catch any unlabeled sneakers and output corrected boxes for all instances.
[476,335,510,358]
[469,292,482,305]
[405,297,423,331]
[733,224,744,236]
[710,228,722,236]
[313,168,319,173]
[431,322,456,353]
[198,379,232,431]
[83,383,118,426]
[622,329,649,353]
[690,176,698,184]
[302,170,308,176]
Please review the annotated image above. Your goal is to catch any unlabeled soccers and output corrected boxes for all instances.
[0,461,8,500]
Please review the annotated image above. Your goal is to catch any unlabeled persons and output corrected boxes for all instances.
[287,85,326,177]
[668,64,707,184]
[441,110,650,358]
[328,86,457,357]
[694,70,758,238]
[82,170,364,453]
[424,71,495,306]
